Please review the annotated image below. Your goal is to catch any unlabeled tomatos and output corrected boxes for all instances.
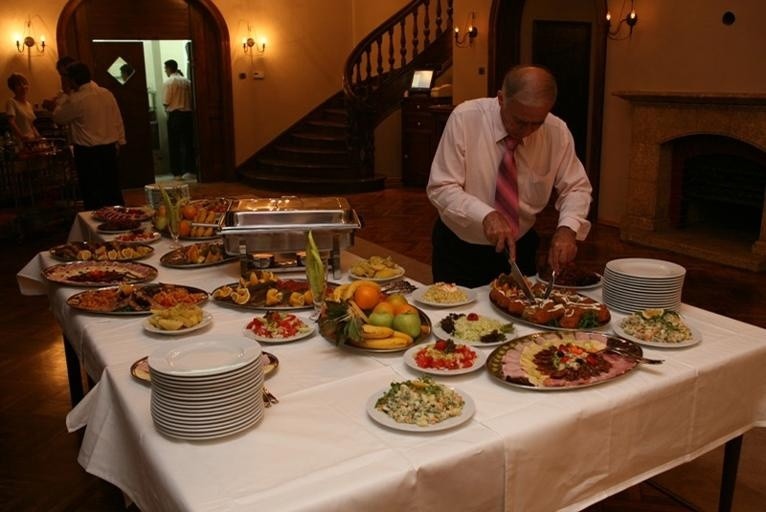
[467,314,478,321]
[435,339,447,350]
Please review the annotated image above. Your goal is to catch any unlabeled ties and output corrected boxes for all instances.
[495,137,520,238]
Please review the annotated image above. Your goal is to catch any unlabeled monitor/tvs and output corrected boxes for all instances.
[408,68,435,94]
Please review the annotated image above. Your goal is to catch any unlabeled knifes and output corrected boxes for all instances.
[500,241,539,305]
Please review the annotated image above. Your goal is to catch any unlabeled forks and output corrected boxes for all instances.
[543,269,561,302]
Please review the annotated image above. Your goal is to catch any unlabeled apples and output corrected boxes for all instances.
[369,311,393,328]
[392,309,421,337]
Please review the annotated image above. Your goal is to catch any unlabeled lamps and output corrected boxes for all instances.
[454,24,477,45]
[603,8,640,36]
[10,23,46,54]
[241,34,268,54]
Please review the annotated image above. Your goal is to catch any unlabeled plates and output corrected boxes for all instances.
[131,349,280,390]
[600,258,686,316]
[210,277,344,311]
[535,266,604,288]
[489,274,610,329]
[89,206,162,247]
[160,240,240,271]
[486,328,645,392]
[50,240,154,263]
[365,377,477,434]
[140,311,214,333]
[350,262,406,281]
[150,334,265,440]
[42,261,159,287]
[610,307,703,354]
[413,283,479,309]
[402,342,486,375]
[316,304,432,354]
[241,315,316,343]
[67,281,209,316]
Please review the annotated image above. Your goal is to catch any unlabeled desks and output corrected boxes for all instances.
[15,206,766,512]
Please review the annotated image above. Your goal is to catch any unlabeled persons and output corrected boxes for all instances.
[6,71,41,139]
[42,56,98,148]
[52,64,128,211]
[162,60,194,170]
[423,63,594,285]
[119,64,133,82]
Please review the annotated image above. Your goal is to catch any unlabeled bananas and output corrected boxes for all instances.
[350,324,414,349]
[332,280,381,303]
